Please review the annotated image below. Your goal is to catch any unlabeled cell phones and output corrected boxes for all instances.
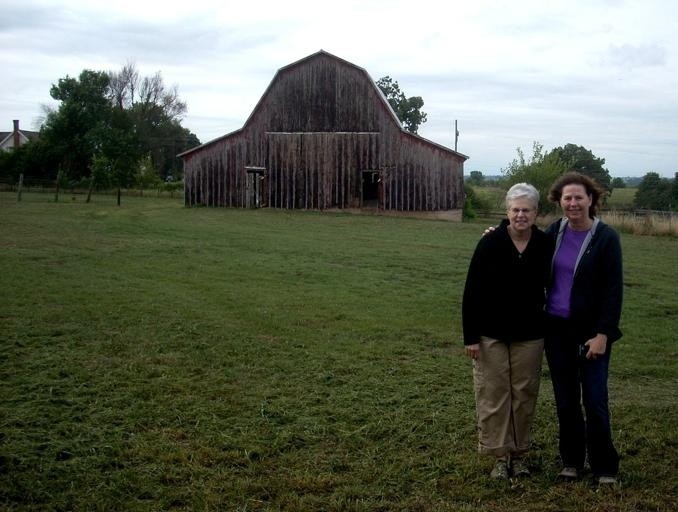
[579,345,590,356]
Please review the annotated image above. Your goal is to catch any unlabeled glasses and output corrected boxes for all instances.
[508,207,536,213]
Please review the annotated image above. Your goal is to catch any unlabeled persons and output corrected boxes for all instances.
[483,173,625,486]
[462,182,549,481]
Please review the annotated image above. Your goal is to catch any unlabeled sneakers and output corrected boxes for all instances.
[488,457,509,480]
[594,475,619,487]
[559,467,580,482]
[510,456,532,478]
